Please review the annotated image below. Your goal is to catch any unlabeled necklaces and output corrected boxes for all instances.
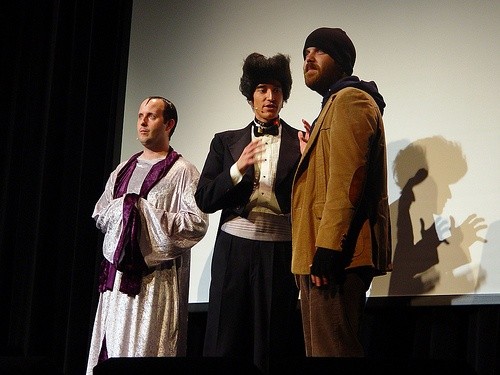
[253,115,281,128]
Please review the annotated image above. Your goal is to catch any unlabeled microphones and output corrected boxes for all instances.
[136,137,139,141]
[249,100,258,111]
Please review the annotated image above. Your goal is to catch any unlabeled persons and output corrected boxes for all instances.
[193,52,313,369]
[289,25,391,357]
[86,95,210,375]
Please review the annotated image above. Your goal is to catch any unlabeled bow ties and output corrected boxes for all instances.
[254,125,279,138]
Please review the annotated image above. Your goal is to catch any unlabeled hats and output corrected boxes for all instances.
[301,25,356,75]
